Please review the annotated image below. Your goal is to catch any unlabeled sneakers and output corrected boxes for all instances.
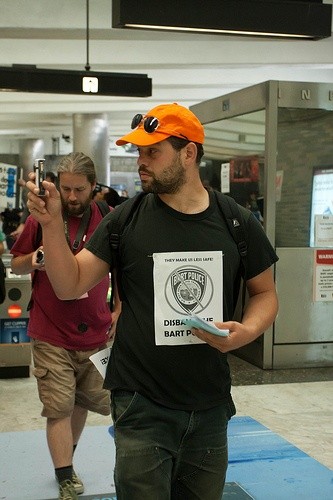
[71,470,85,494]
[58,478,79,500]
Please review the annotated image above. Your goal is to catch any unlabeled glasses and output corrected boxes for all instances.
[131,114,189,141]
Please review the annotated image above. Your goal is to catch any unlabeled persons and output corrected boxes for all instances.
[24,102,279,500]
[10,152,126,500]
[1,182,267,249]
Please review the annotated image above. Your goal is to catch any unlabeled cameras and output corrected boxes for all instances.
[33,159,46,197]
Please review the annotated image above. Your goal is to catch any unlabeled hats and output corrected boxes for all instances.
[116,103,205,146]
